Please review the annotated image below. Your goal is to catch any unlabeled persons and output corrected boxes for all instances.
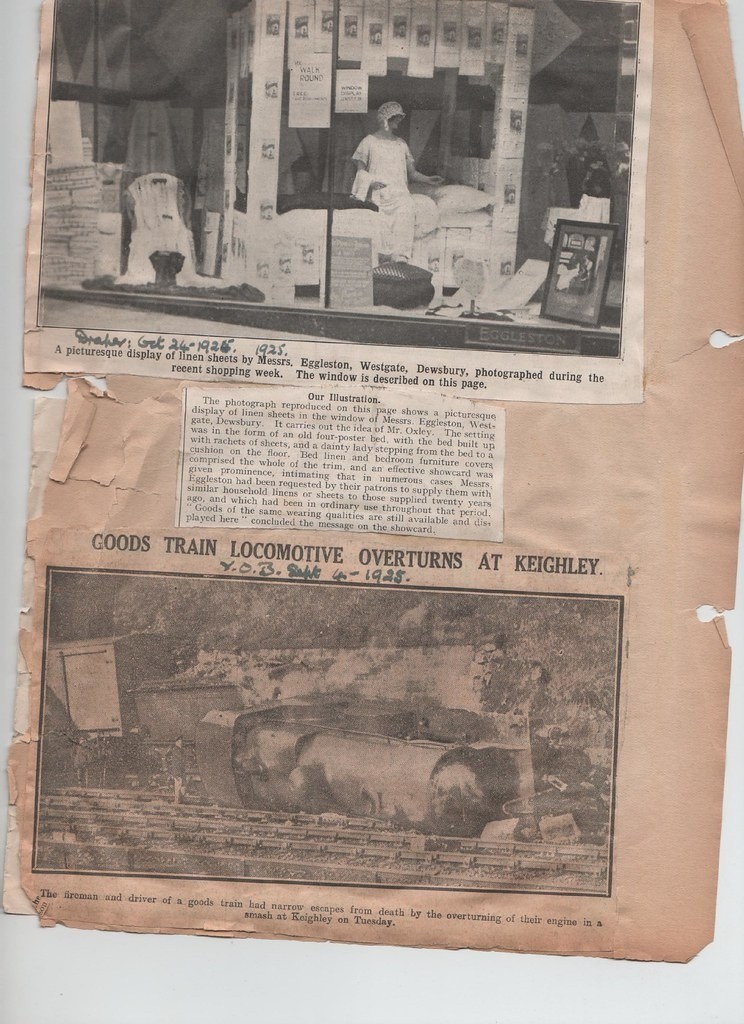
[352,100,444,264]
[170,735,189,805]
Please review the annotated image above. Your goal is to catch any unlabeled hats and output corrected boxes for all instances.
[615,142,631,154]
[377,100,406,131]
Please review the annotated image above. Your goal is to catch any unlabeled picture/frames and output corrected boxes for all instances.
[539,216,620,329]
